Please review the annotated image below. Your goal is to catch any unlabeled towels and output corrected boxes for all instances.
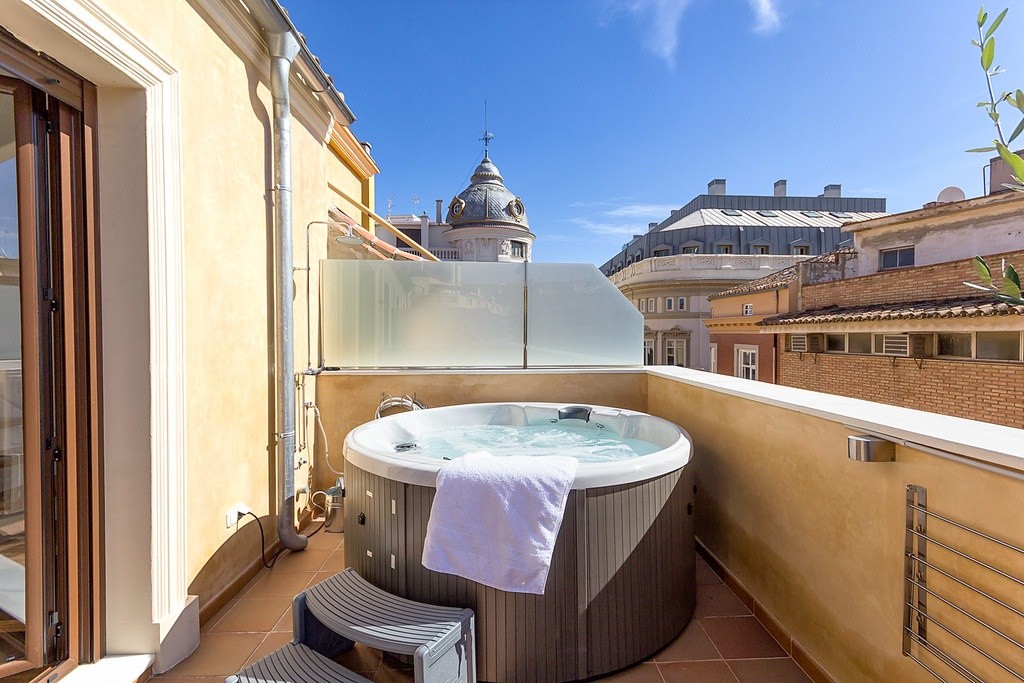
[422,450,579,595]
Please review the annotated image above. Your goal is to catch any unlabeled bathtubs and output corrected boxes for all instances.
[340,402,698,682]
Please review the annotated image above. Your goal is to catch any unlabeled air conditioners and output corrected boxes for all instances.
[790,335,823,352]
[883,334,925,358]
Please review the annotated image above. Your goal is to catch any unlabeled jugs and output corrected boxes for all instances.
[311,487,345,533]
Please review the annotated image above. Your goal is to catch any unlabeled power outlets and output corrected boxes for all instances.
[225,501,251,527]
